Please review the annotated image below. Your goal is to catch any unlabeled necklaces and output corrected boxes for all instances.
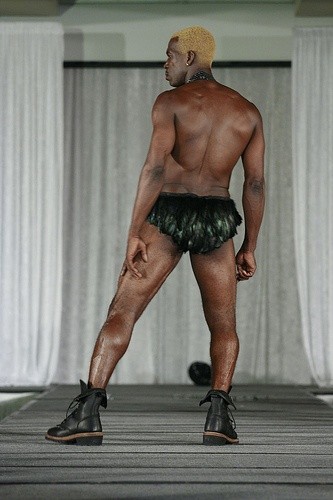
[185,71,215,84]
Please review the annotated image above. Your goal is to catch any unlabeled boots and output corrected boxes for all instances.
[200,386,239,444]
[44,379,108,446]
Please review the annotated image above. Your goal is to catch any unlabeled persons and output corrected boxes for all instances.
[45,24,265,446]
